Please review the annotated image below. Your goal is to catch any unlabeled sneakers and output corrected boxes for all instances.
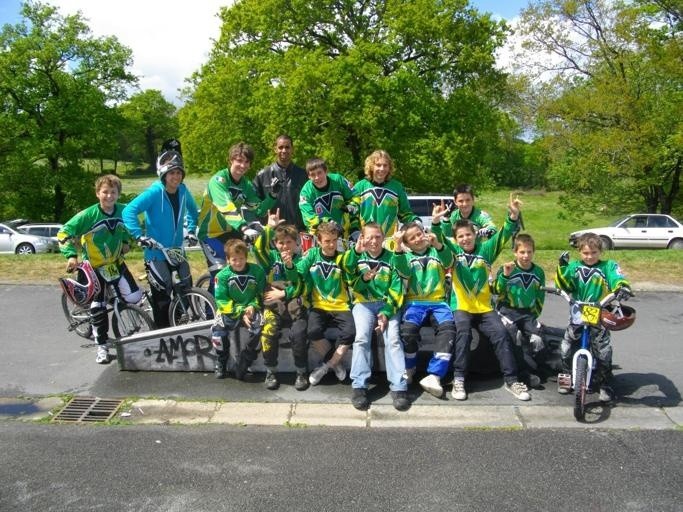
[504,380,530,401]
[451,379,467,401]
[236,349,257,380]
[215,356,226,379]
[598,385,613,403]
[559,374,572,394]
[404,365,416,385]
[419,374,444,398]
[390,390,412,412]
[264,366,281,390]
[96,344,110,364]
[295,367,308,391]
[351,387,370,412]
[531,374,540,389]
[309,357,347,387]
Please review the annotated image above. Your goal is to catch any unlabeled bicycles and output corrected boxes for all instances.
[60,220,304,354]
[533,284,637,421]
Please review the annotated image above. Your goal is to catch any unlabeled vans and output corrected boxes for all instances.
[397,193,502,244]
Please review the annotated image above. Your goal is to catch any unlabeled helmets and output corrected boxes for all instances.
[156,149,186,181]
[600,303,636,331]
[58,259,101,306]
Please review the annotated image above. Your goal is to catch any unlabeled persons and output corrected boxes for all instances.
[295,156,360,242]
[122,152,196,329]
[197,142,283,320]
[206,182,546,411]
[345,150,425,236]
[56,173,142,365]
[551,233,630,403]
[250,134,308,233]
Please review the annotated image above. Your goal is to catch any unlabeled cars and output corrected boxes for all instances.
[0,216,70,256]
[566,209,682,251]
[179,207,202,243]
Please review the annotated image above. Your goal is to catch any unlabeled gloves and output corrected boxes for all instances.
[616,286,633,302]
[558,250,571,266]
[443,201,456,218]
[349,228,361,243]
[478,227,496,238]
[343,205,357,216]
[270,177,284,198]
[242,226,259,244]
[183,233,198,247]
[137,235,157,251]
[414,219,425,230]
